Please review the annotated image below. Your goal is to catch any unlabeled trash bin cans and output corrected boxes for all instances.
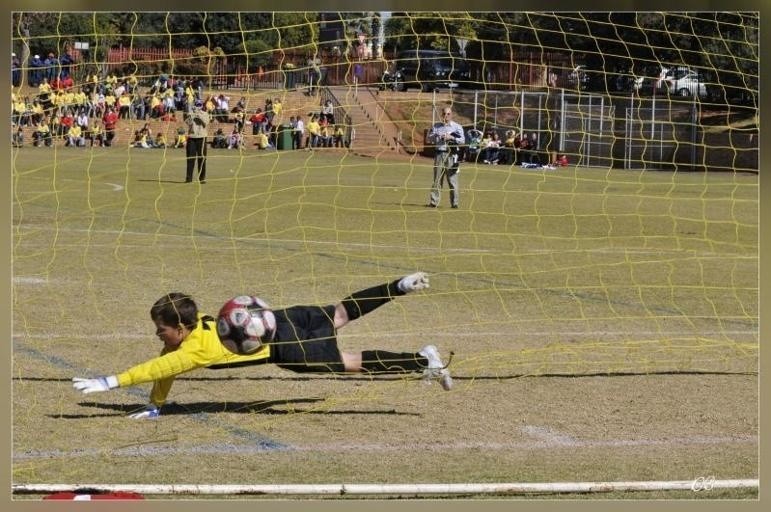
[279,125,294,150]
[424,129,436,157]
[283,65,297,88]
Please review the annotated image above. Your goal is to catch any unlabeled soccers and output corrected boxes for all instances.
[216,295,277,355]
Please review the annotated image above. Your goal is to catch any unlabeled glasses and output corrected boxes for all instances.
[443,113,450,115]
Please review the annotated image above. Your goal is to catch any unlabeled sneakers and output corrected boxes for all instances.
[430,204,437,208]
[397,272,430,291]
[418,344,452,391]
[452,205,458,209]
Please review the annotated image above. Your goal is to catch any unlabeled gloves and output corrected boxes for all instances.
[72,376,110,394]
[124,403,162,420]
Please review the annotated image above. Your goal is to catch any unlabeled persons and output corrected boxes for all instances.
[11,51,355,150]
[467,128,538,166]
[426,106,465,208]
[72,271,453,419]
[183,100,209,184]
